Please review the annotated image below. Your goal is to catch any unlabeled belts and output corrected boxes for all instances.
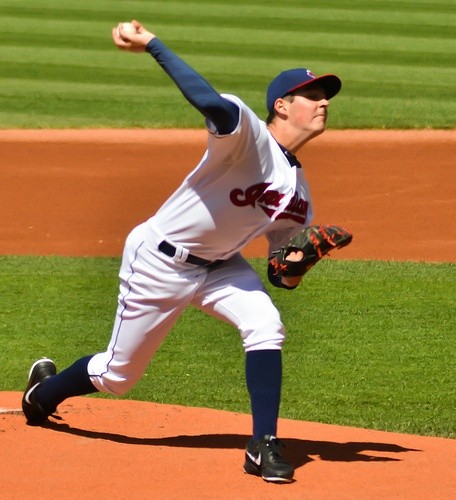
[160,240,220,268]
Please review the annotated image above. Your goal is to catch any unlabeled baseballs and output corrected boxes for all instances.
[117,22,137,36]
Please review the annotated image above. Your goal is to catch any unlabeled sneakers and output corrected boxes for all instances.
[242,436,295,482]
[21,358,57,425]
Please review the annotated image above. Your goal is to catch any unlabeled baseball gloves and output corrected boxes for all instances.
[266,226,352,279]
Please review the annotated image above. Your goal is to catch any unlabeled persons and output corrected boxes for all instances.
[22,19,352,483]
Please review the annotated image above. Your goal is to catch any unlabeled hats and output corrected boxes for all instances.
[266,67,342,112]
